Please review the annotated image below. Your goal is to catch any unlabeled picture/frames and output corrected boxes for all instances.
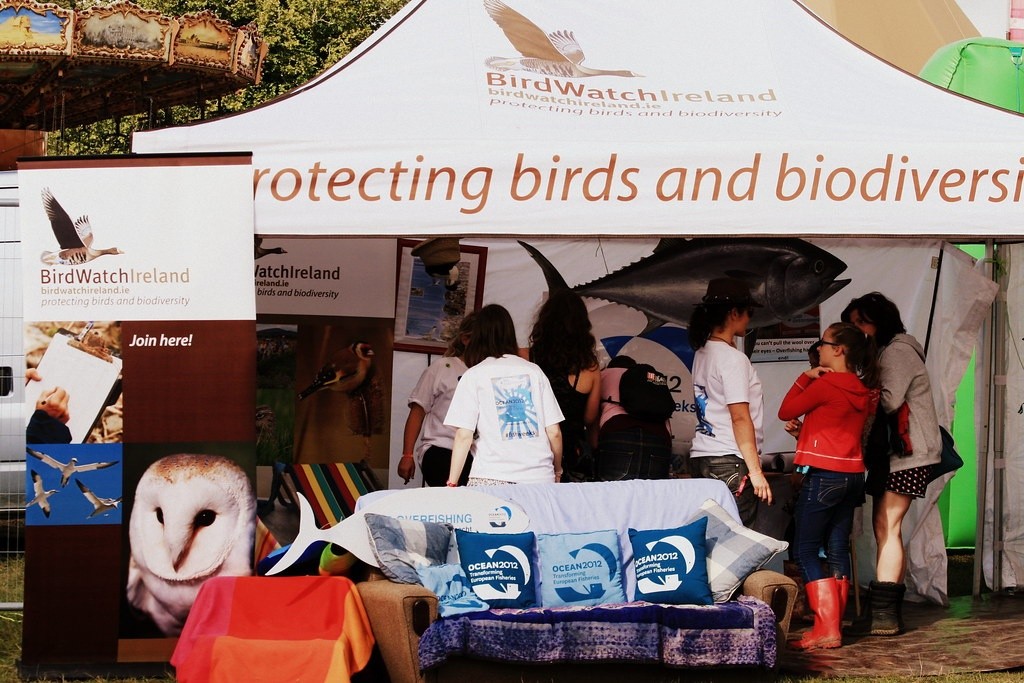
[392,238,488,355]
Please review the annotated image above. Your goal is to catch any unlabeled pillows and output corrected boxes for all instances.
[415,564,490,617]
[538,529,626,609]
[628,516,714,605]
[364,513,452,584]
[454,528,541,609]
[257,540,328,576]
[677,498,789,603]
[318,542,360,577]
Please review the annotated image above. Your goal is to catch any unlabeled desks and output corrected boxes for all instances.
[670,472,861,626]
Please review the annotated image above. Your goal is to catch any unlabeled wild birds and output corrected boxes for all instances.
[26,469,61,519]
[26,447,119,489]
[298,336,374,399]
[40,186,124,267]
[74,478,122,520]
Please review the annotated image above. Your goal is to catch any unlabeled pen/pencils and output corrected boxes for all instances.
[404,475,410,485]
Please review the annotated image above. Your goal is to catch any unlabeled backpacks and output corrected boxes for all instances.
[598,364,676,427]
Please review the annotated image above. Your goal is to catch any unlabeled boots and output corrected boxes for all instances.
[802,576,850,640]
[843,579,907,635]
[787,578,842,648]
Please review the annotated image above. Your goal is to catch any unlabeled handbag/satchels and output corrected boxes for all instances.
[926,425,964,485]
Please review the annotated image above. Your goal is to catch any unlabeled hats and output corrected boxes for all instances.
[702,276,764,308]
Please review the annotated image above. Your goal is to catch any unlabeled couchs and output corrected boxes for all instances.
[351,479,799,683]
[171,577,374,683]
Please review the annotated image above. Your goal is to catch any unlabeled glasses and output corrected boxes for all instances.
[820,338,845,355]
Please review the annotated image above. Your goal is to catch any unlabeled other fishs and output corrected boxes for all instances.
[518,238,852,359]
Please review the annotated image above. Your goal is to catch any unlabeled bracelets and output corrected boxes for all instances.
[446,481,458,487]
[794,382,805,390]
[401,453,413,458]
[555,468,563,475]
[747,472,762,477]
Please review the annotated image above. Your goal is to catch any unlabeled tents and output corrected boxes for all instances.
[132,0,1024,619]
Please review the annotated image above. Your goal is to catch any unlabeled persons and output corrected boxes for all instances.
[397,313,479,487]
[25,355,73,444]
[777,322,889,650]
[446,304,565,487]
[527,287,601,483]
[589,356,672,482]
[840,291,942,635]
[690,277,773,531]
